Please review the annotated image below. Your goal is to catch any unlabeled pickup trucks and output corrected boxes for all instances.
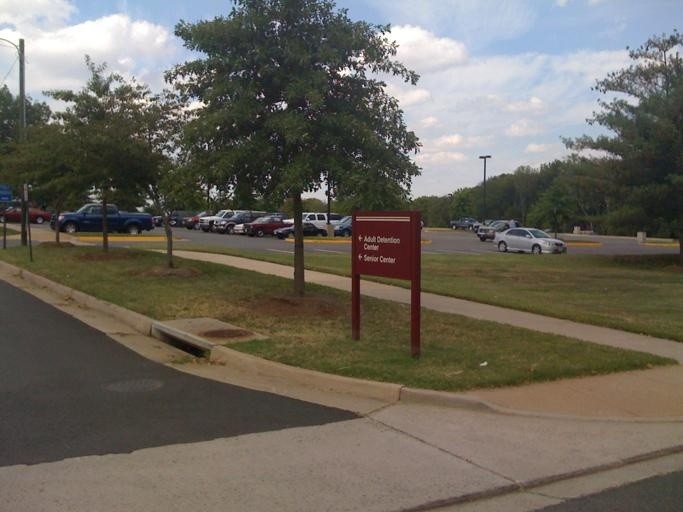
[50,203,154,235]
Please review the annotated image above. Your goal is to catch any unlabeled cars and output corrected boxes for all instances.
[0,205,52,225]
[450,217,568,255]
[153,203,352,239]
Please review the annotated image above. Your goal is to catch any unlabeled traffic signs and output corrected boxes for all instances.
[354,212,411,280]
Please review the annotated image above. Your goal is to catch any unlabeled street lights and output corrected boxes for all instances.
[479,155,491,208]
